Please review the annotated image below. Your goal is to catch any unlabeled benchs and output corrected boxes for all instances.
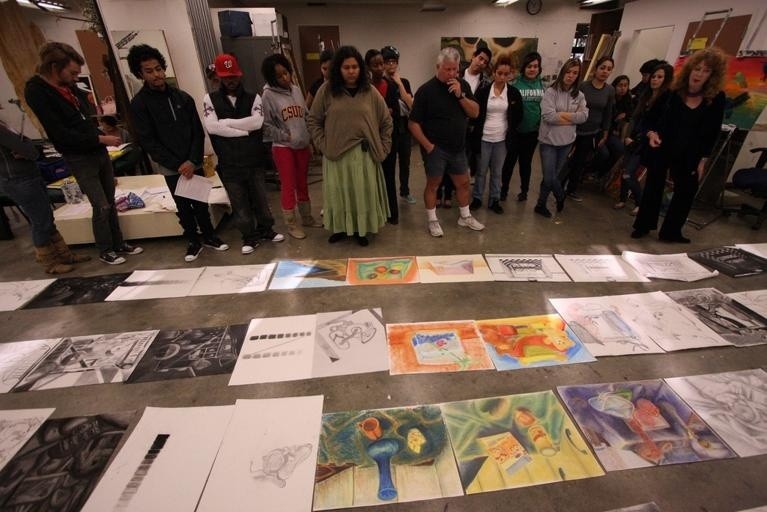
[50,173,229,246]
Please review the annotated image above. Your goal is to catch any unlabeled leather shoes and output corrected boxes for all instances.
[630,207,639,216]
[329,232,342,243]
[355,232,368,246]
[614,201,626,210]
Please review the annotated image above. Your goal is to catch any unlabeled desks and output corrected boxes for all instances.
[0,147,145,226]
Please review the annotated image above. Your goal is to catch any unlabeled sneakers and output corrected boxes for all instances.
[184,241,203,262]
[257,230,284,242]
[241,240,260,253]
[98,251,126,264]
[426,220,444,237]
[201,234,229,250]
[457,214,486,230]
[113,242,144,255]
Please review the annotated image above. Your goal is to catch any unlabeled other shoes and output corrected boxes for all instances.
[434,199,443,209]
[399,193,415,203]
[517,194,527,202]
[443,199,452,209]
[630,229,651,239]
[534,204,552,218]
[488,199,503,214]
[500,192,508,201]
[555,192,568,212]
[659,234,690,244]
[470,197,483,212]
[567,191,583,202]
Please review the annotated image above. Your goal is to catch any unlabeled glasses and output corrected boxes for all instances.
[386,59,396,65]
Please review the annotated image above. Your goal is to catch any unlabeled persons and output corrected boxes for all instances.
[0,124,90,274]
[127,44,229,262]
[24,42,143,266]
[99,115,137,176]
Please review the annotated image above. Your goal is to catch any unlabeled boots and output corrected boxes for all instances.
[296,199,324,227]
[281,206,306,238]
[55,237,90,264]
[35,243,73,274]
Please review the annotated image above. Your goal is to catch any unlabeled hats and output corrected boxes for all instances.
[215,54,243,77]
[206,63,215,77]
[381,45,399,59]
